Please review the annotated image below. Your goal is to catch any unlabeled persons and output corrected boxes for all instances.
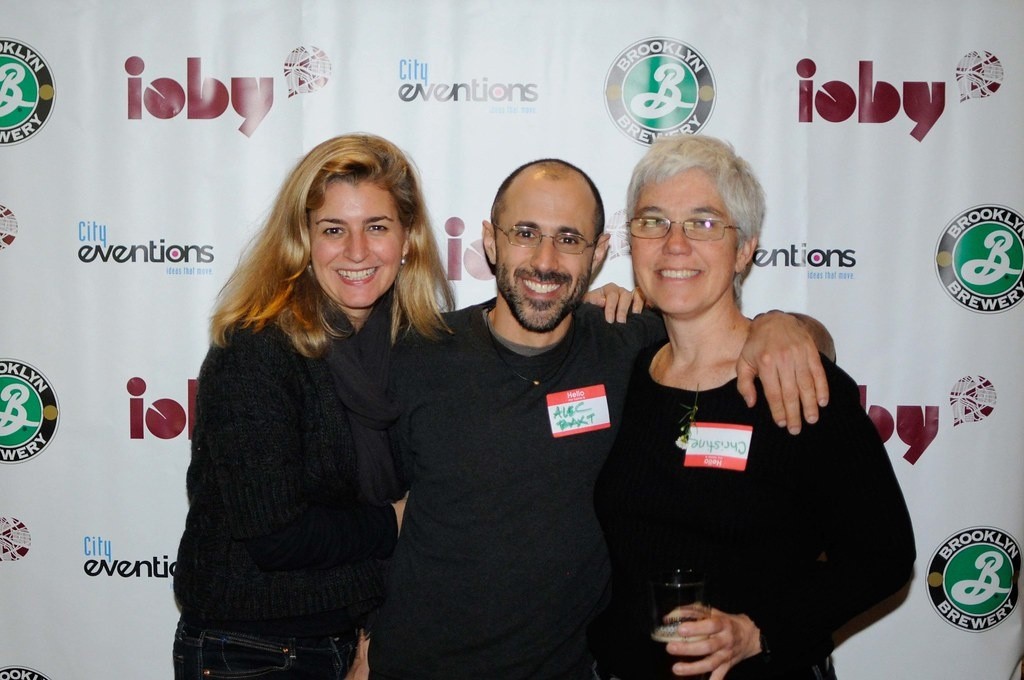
[348,156,832,679]
[172,133,646,680]
[596,131,917,678]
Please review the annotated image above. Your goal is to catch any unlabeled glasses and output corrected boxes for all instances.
[493,220,604,254]
[627,217,740,241]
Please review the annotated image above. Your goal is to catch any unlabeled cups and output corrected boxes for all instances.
[649,571,706,638]
